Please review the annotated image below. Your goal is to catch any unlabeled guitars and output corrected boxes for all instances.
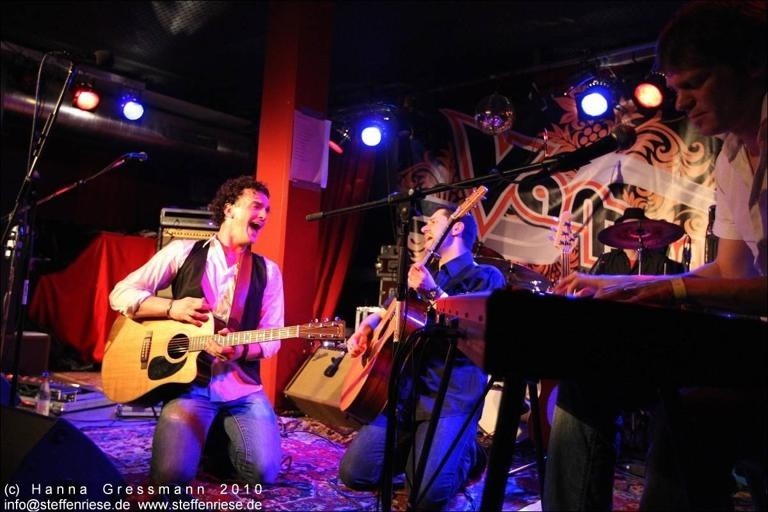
[341,185,487,425]
[101,305,346,406]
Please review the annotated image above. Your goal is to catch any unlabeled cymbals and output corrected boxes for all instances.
[599,218,685,250]
[473,257,551,286]
[472,243,501,258]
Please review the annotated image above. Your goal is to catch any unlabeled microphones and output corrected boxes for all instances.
[127,152,149,163]
[324,348,346,377]
[544,125,637,173]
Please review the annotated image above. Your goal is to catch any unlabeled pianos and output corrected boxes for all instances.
[481,278,767,431]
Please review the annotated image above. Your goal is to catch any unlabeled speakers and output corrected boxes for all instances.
[157,207,218,248]
[1,404,131,511]
[284,348,386,433]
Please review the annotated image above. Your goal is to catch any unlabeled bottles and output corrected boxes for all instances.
[35,372,50,417]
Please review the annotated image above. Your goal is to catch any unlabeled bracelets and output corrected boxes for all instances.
[167,300,174,319]
[671,277,687,299]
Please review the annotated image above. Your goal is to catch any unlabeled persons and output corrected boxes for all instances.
[540,1,768,512]
[338,207,507,502]
[108,176,285,486]
